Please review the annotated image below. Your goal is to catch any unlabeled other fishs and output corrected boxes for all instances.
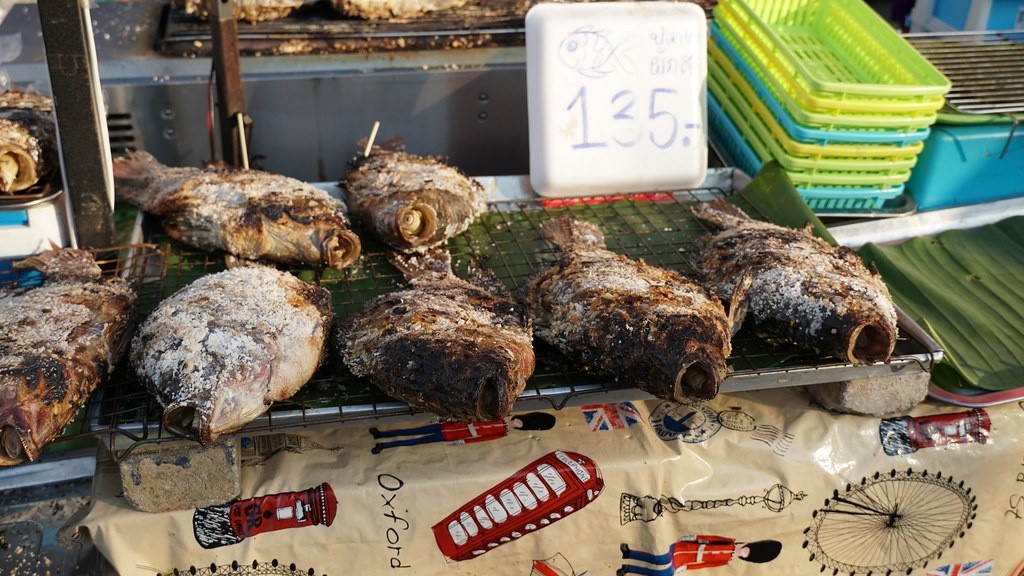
[0,90,900,464]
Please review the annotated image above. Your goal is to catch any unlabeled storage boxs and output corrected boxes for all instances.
[705,0,954,211]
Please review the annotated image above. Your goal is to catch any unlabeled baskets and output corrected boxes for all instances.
[708,0,952,211]
